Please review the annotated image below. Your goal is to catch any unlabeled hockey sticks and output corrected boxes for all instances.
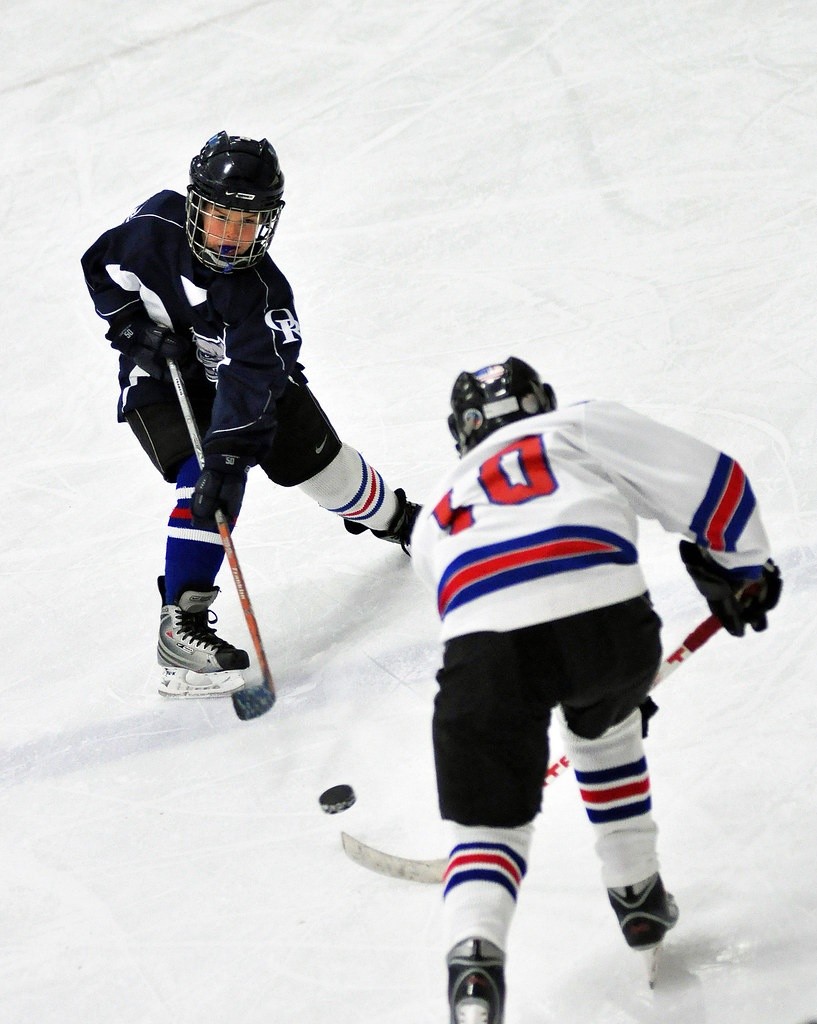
[342,557,776,886]
[162,359,280,727]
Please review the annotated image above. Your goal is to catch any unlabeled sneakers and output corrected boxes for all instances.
[445,935,505,1024]
[344,489,423,558]
[157,577,251,698]
[606,869,678,992]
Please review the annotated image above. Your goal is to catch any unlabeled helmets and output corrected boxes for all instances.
[187,131,286,270]
[447,356,557,458]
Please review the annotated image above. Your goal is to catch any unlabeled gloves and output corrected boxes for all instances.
[104,317,192,375]
[680,541,783,635]
[190,437,254,532]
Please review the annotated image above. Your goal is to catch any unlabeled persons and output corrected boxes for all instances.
[81,131,422,698]
[410,356,784,1024]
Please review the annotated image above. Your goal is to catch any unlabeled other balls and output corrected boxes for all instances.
[320,784,355,813]
